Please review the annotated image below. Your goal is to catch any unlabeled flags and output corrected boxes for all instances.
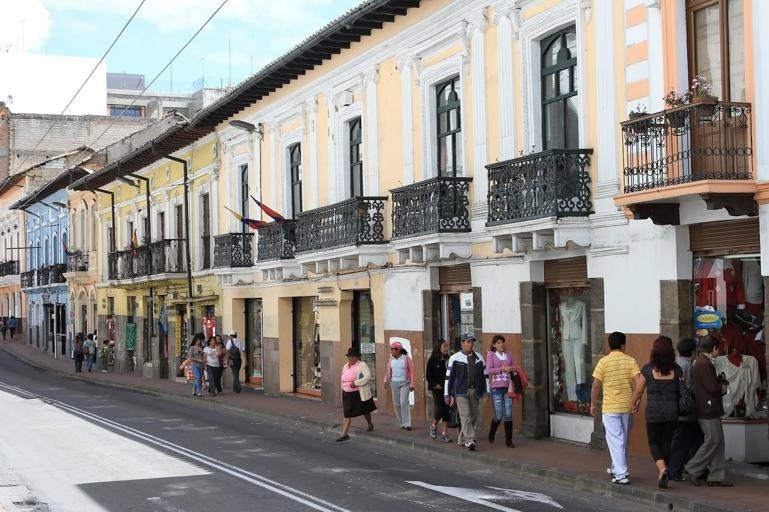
[628,111,652,133]
[664,107,687,128]
[691,94,720,117]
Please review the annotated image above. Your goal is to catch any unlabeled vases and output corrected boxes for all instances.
[690,71,716,97]
[662,89,688,108]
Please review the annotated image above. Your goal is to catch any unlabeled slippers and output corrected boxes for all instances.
[97,344,107,360]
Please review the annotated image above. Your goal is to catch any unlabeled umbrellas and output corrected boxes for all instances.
[198,394,202,397]
[191,393,196,396]
[707,480,734,488]
[366,424,376,432]
[336,434,350,441]
[684,468,701,486]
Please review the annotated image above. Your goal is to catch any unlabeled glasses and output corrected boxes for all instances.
[229,120,265,141]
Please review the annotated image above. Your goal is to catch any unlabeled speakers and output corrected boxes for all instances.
[488,418,502,444]
[504,421,516,449]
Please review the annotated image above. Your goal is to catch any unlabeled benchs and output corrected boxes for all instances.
[131,234,139,258]
[225,206,266,229]
[254,199,285,221]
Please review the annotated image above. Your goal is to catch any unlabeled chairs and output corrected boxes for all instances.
[611,477,630,484]
[671,475,686,481]
[606,467,630,476]
[209,391,224,397]
[441,431,453,443]
[658,468,669,490]
[400,425,413,432]
[465,440,477,448]
[429,424,438,439]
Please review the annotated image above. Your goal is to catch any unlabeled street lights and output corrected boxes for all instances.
[83,338,89,355]
[358,383,374,402]
[229,339,241,361]
[675,364,696,418]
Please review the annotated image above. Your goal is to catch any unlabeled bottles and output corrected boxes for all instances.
[229,331,238,336]
[344,347,362,357]
[390,342,403,351]
[460,334,477,342]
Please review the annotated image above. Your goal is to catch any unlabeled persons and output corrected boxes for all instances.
[559,297,588,403]
[426,339,453,444]
[487,335,521,447]
[384,342,416,430]
[590,332,733,491]
[443,334,490,450]
[189,330,248,397]
[0,315,16,340]
[73,331,115,373]
[336,348,377,442]
[692,257,764,419]
